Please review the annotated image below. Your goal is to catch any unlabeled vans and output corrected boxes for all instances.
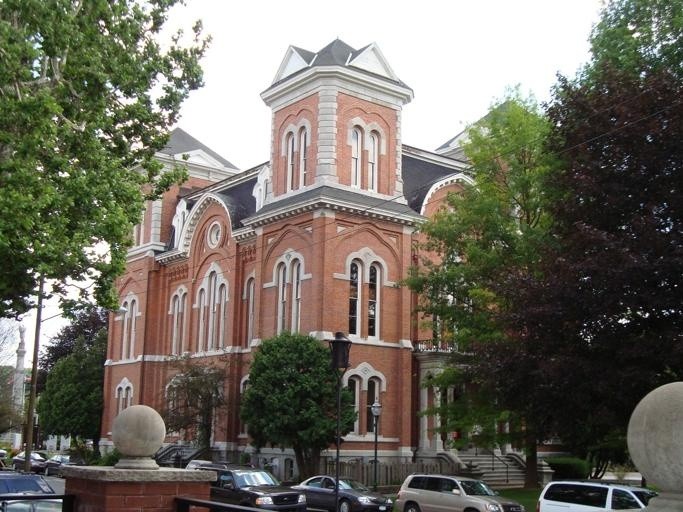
[535,478,651,512]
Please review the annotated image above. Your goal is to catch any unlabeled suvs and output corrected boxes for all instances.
[394,471,525,512]
[181,458,311,512]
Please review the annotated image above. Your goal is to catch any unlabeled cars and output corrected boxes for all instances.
[287,472,393,511]
[0,447,86,512]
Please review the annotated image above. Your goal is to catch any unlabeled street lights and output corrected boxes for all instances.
[368,395,384,492]
[327,325,357,512]
